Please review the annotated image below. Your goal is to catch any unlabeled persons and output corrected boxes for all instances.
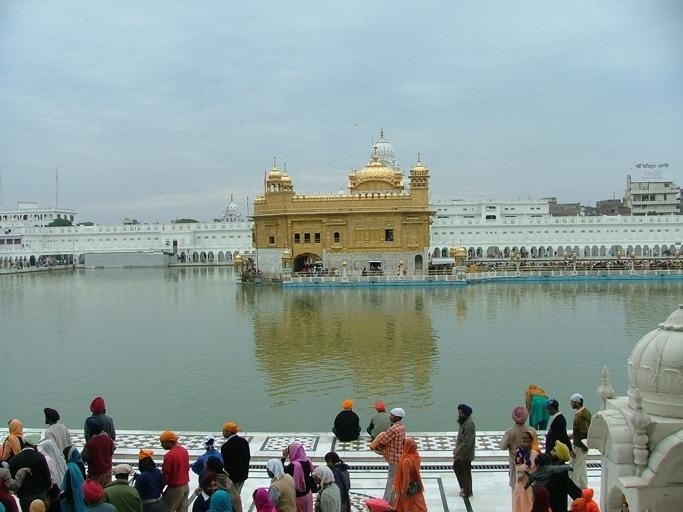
[450,403,475,498]
[523,453,599,512]
[566,393,592,489]
[0,254,78,276]
[524,383,552,429]
[500,406,542,492]
[512,430,540,512]
[544,398,577,462]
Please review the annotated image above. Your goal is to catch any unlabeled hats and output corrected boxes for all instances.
[512,406,529,424]
[457,404,472,415]
[112,463,132,475]
[82,479,105,502]
[20,433,41,446]
